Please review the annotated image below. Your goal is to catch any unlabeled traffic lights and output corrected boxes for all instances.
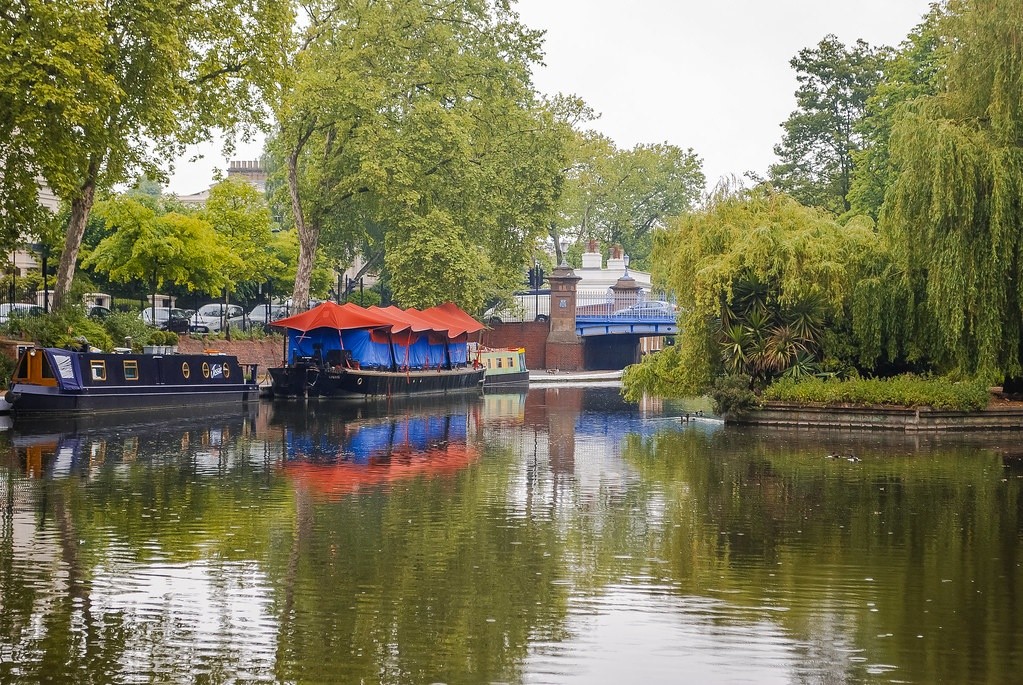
[540,269,545,286]
[528,268,535,285]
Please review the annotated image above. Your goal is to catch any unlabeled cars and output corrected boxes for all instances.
[188,303,248,334]
[0,302,47,326]
[613,300,683,318]
[138,306,189,334]
[183,309,197,318]
[80,305,112,321]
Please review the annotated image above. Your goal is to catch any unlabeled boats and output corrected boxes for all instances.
[4,345,260,410]
[465,341,530,384]
[266,301,491,398]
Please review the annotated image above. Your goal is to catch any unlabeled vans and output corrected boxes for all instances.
[248,305,286,334]
[483,294,551,322]
[285,298,320,316]
